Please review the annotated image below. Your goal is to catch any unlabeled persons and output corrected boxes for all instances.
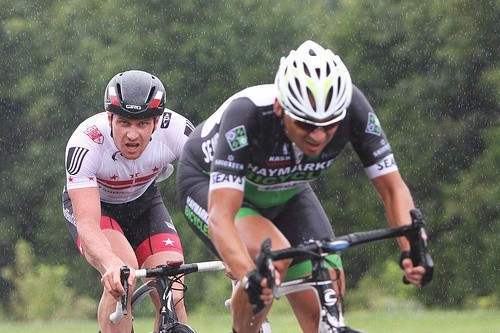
[176,39,435,333]
[61,69,195,333]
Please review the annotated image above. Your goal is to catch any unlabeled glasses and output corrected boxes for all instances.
[290,119,340,132]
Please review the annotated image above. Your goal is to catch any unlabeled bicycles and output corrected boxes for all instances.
[249,207,431,333]
[96,258,236,333]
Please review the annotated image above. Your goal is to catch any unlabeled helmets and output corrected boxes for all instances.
[275,40,353,127]
[104,70,166,119]
